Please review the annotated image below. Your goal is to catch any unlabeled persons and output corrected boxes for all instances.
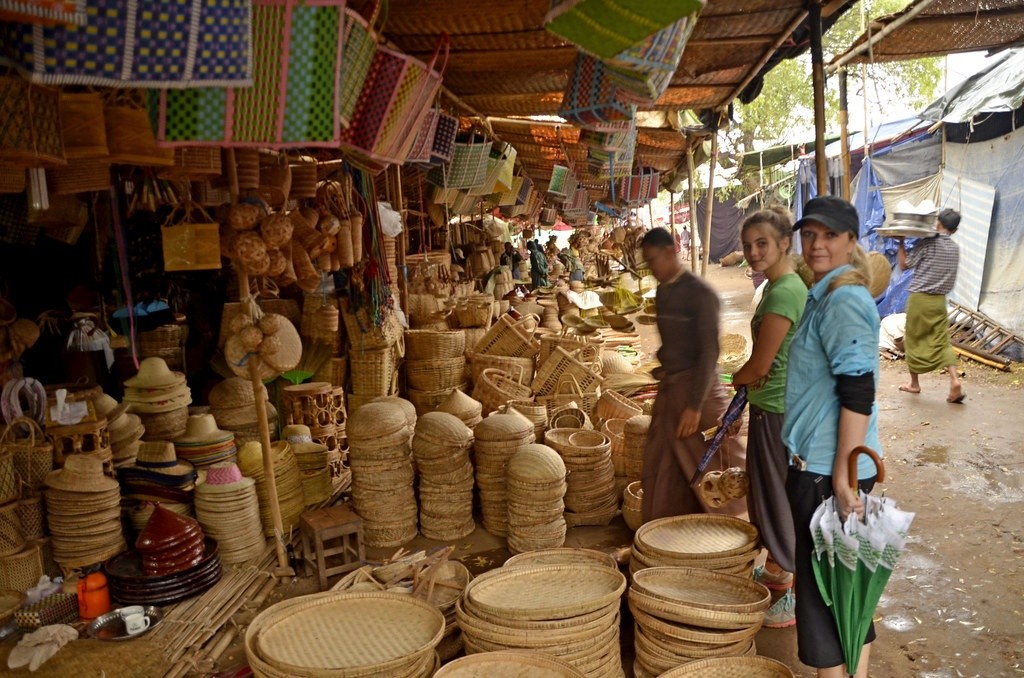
[616,205,809,628]
[681,226,691,261]
[526,235,585,291]
[779,197,881,678]
[455,248,465,260]
[500,242,523,279]
[892,209,967,402]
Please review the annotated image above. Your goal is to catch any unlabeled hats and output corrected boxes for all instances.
[45,356,334,575]
[938,208,960,232]
[791,194,859,238]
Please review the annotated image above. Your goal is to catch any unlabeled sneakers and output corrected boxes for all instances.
[761,587,799,628]
[753,564,794,590]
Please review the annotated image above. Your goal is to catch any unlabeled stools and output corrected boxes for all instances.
[298,504,365,593]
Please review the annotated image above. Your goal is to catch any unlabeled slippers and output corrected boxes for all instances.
[946,392,965,402]
[899,384,920,393]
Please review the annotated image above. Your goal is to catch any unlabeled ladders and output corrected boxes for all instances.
[943,299,1024,373]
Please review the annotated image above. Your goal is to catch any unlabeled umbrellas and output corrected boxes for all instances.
[809,444,916,678]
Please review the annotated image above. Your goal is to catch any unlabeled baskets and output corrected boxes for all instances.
[338,286,748,557]
[0,0,708,284]
[0,416,80,633]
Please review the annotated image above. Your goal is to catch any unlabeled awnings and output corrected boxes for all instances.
[732,130,861,210]
[824,0,1024,201]
[345,0,861,280]
